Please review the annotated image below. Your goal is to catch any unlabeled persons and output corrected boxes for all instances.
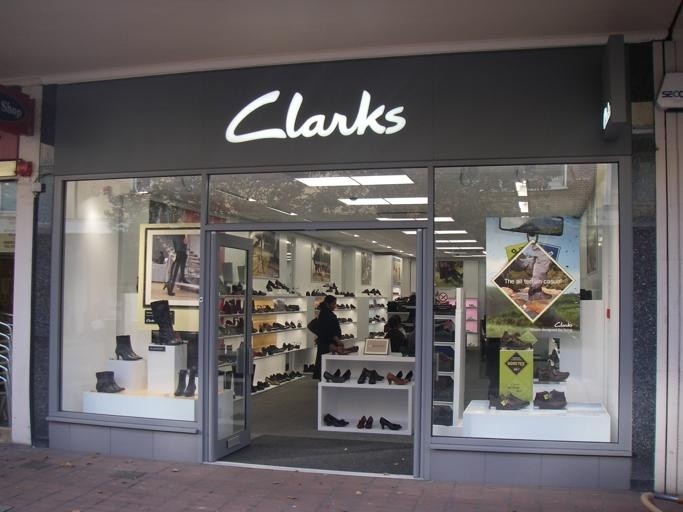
[483,338,500,400]
[312,295,343,384]
[440,261,462,287]
[162,208,189,297]
[385,314,407,351]
[313,243,323,276]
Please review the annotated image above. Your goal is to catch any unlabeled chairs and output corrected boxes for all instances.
[0,307,13,428]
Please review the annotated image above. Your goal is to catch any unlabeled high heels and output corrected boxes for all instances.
[357,416,373,429]
[380,417,401,430]
[330,342,351,356]
[323,368,351,383]
[387,370,413,386]
[324,413,349,427]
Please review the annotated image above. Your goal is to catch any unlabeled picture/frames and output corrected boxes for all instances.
[140,225,200,308]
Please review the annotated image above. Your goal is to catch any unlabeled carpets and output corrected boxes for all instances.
[218,433,414,476]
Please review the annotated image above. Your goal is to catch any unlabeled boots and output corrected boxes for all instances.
[163,262,180,296]
[174,368,196,397]
[115,335,143,361]
[178,261,190,284]
[96,371,125,393]
[150,300,184,346]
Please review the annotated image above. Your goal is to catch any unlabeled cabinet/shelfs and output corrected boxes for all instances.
[445,297,480,349]
[310,295,359,369]
[358,294,387,342]
[217,294,307,401]
[316,353,418,437]
[386,286,466,426]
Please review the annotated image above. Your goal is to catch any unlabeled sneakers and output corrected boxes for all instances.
[537,366,570,382]
[528,288,553,301]
[489,392,531,411]
[358,368,384,384]
[501,331,532,351]
[304,282,387,373]
[217,279,303,399]
[534,389,568,409]
